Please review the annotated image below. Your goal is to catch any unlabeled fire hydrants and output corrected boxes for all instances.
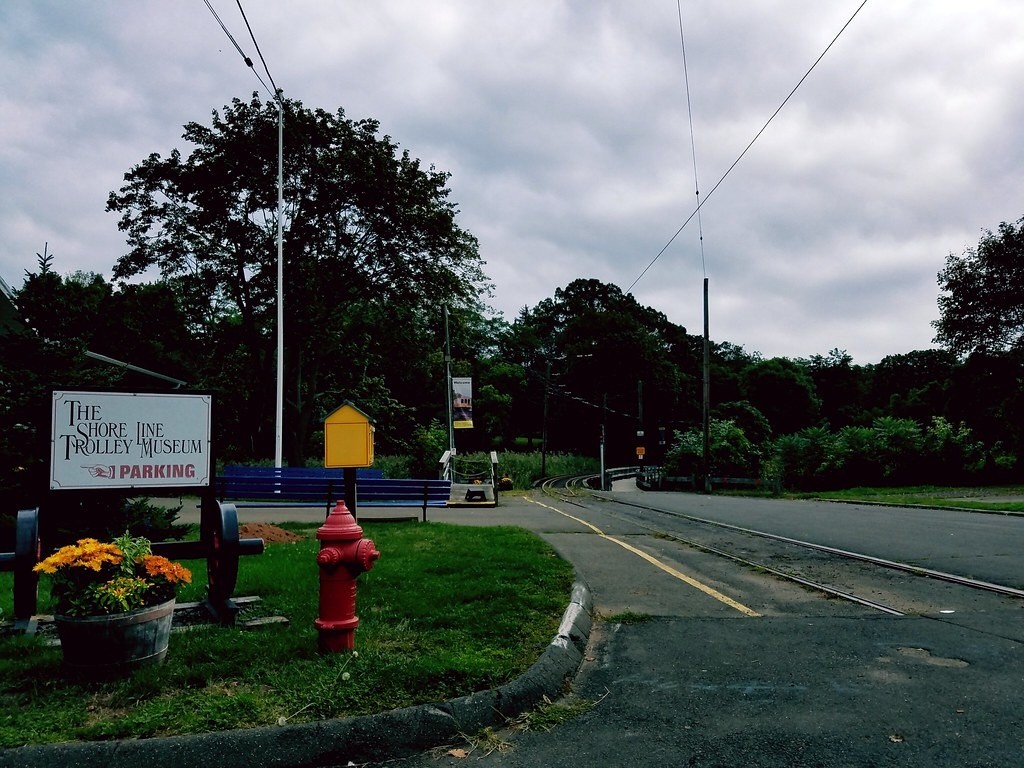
[315,499,379,652]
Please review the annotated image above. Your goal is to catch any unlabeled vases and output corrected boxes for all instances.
[53,598,174,684]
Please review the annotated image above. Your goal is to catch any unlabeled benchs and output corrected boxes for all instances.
[197,468,450,521]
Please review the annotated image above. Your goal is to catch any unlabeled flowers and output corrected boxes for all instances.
[32,537,193,617]
